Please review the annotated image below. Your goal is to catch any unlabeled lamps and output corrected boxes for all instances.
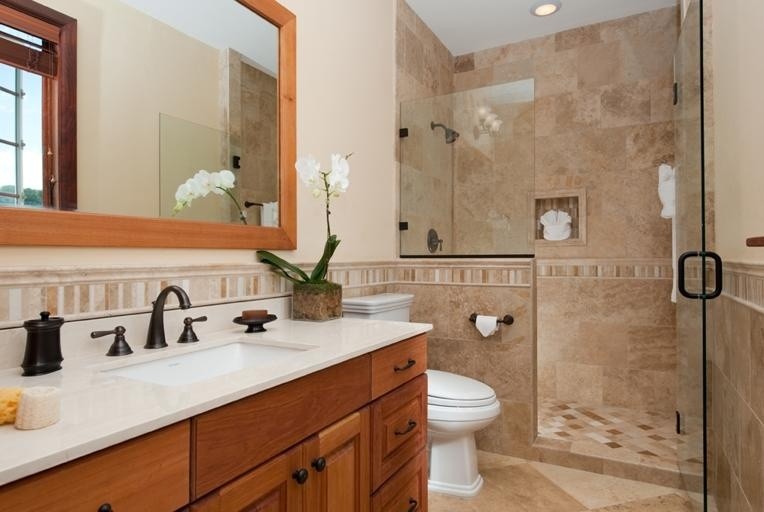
[474,106,503,141]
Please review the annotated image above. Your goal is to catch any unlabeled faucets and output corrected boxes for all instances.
[144,285,192,349]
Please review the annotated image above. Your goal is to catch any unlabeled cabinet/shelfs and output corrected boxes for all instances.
[369,333,429,510]
[0,420,189,512]
[189,353,369,510]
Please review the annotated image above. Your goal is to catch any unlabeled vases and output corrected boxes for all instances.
[291,284,341,322]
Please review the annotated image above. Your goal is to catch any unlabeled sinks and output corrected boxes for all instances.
[94,336,318,391]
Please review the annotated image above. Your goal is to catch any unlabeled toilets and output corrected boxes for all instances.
[340,292,501,498]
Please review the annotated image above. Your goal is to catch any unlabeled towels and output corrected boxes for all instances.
[539,209,572,242]
[258,202,279,228]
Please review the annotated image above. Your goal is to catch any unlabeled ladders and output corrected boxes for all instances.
[0,62,26,207]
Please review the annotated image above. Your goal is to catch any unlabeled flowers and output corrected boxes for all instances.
[170,166,249,226]
[254,153,352,284]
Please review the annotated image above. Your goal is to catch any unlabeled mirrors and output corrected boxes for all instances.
[0,0,296,251]
